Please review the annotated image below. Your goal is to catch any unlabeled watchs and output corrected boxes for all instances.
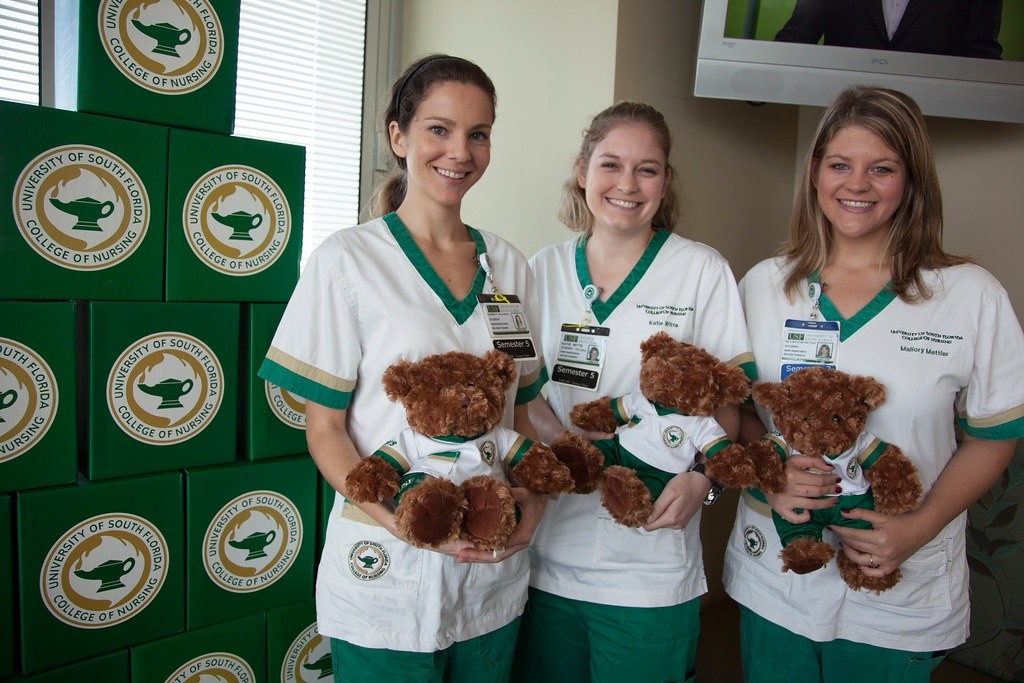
[689,462,721,505]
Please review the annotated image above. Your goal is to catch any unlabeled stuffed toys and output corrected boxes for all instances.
[748,366,921,595]
[343,354,575,550]
[548,331,760,528]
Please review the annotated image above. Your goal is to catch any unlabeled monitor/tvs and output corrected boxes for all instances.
[692,0,1024,127]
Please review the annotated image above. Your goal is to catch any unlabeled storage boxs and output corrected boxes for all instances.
[0,1,337,683]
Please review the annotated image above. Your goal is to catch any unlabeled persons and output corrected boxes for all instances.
[721,86,1024,683]
[515,104,760,683]
[588,347,599,360]
[817,345,831,358]
[773,0,1004,60]
[516,315,524,326]
[256,54,551,683]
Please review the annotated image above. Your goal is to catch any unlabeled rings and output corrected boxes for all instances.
[870,554,873,564]
